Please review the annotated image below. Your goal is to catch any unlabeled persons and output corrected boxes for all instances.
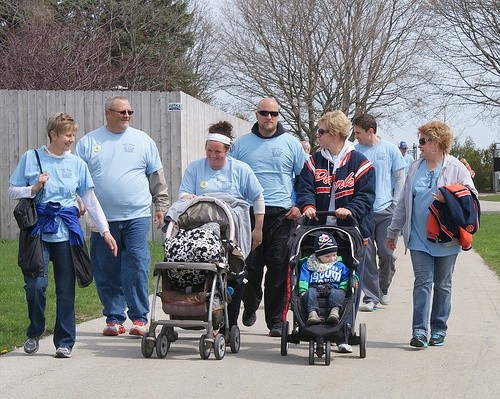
[398,141,414,165]
[296,110,376,353]
[352,114,408,312]
[74,97,170,336]
[8,113,118,358]
[299,232,351,326]
[300,141,312,157]
[229,98,308,337]
[386,122,481,348]
[179,121,265,346]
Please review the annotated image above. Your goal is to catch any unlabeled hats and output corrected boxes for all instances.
[398,140,409,149]
[313,231,337,257]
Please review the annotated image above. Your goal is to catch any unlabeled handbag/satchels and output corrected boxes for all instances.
[13,148,44,232]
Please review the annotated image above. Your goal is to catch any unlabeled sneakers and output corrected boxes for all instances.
[54,342,76,358]
[242,289,263,327]
[379,285,391,306]
[409,328,430,349]
[128,320,150,336]
[269,327,283,337]
[24,328,47,355]
[103,323,125,336]
[357,301,377,312]
[337,343,353,353]
[429,330,447,346]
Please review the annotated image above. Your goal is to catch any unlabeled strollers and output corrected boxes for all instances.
[139,196,241,359]
[279,210,370,367]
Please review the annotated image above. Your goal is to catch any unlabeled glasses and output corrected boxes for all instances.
[109,107,133,116]
[425,170,435,189]
[317,129,330,136]
[418,138,433,145]
[258,111,279,117]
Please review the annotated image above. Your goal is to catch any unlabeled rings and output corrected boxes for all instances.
[259,242,262,245]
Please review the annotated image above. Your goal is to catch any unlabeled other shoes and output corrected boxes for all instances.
[306,312,321,325]
[326,311,340,324]
